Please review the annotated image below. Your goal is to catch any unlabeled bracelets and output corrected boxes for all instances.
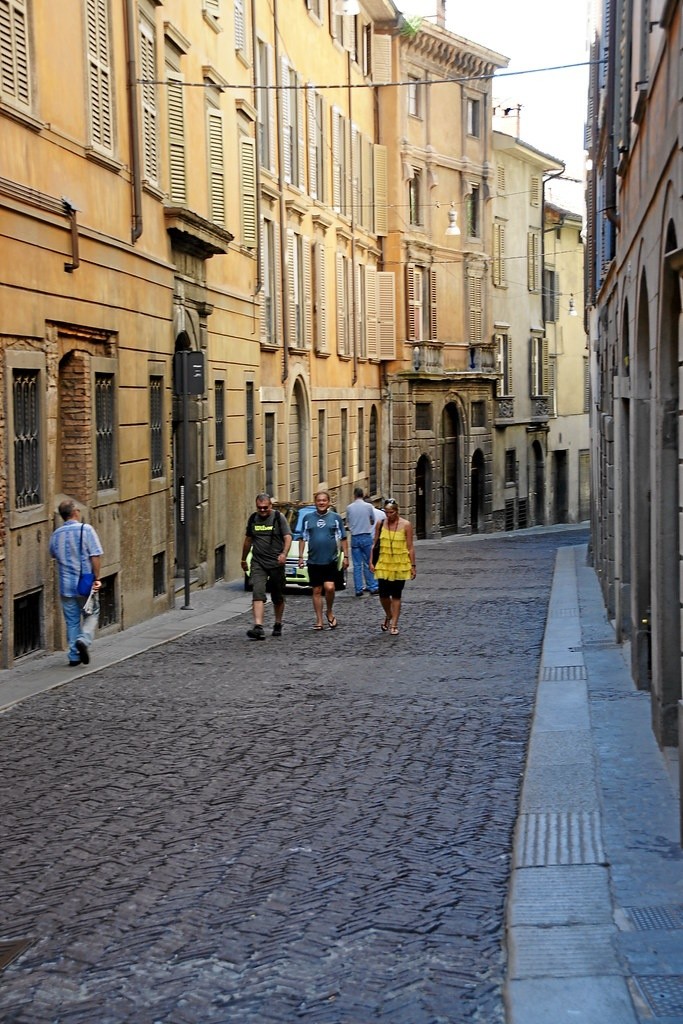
[344,556,348,558]
[412,565,416,567]
[240,560,246,563]
[299,557,303,558]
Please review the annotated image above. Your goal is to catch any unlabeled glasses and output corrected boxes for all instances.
[384,499,397,506]
[256,504,270,509]
[73,508,80,513]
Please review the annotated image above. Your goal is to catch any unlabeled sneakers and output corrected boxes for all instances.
[272,622,283,636]
[247,625,265,641]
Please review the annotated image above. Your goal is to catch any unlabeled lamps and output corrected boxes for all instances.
[568,298,578,316]
[445,203,462,236]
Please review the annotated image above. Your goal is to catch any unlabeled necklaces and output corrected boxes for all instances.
[388,519,398,550]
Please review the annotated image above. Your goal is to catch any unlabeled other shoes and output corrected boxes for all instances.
[68,659,81,666]
[362,586,370,591]
[355,591,363,596]
[370,589,378,596]
[75,641,89,664]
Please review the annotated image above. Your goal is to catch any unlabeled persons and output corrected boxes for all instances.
[298,492,350,630]
[49,499,104,667]
[346,487,386,596]
[241,493,293,640]
[369,499,416,635]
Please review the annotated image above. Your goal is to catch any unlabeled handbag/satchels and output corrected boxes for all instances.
[78,573,94,596]
[372,518,384,567]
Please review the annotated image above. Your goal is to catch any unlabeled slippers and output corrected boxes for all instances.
[313,624,323,631]
[390,626,399,635]
[381,615,391,630]
[326,613,337,628]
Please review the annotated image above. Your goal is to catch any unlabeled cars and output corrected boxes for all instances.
[244,501,351,593]
